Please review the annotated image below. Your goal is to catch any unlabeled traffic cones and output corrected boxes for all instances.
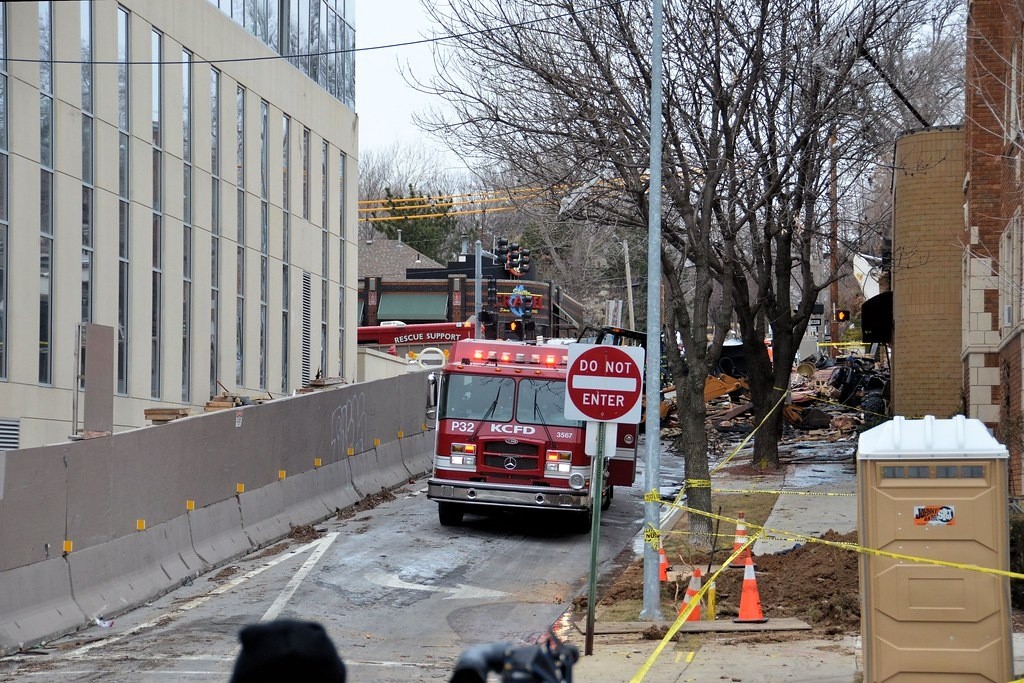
[734,559,770,624]
[661,540,674,571]
[676,566,703,621]
[728,508,760,568]
[660,542,670,581]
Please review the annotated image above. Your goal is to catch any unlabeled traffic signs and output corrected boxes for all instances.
[809,318,821,326]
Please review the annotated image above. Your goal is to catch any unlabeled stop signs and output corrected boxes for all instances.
[565,346,642,421]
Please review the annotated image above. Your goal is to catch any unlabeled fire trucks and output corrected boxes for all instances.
[424,339,643,535]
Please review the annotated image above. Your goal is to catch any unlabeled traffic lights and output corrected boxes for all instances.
[508,242,520,268]
[478,310,490,323]
[836,309,851,322]
[497,237,509,263]
[521,295,533,318]
[520,247,530,273]
[509,322,521,332]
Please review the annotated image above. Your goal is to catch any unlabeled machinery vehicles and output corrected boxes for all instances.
[575,323,831,434]
